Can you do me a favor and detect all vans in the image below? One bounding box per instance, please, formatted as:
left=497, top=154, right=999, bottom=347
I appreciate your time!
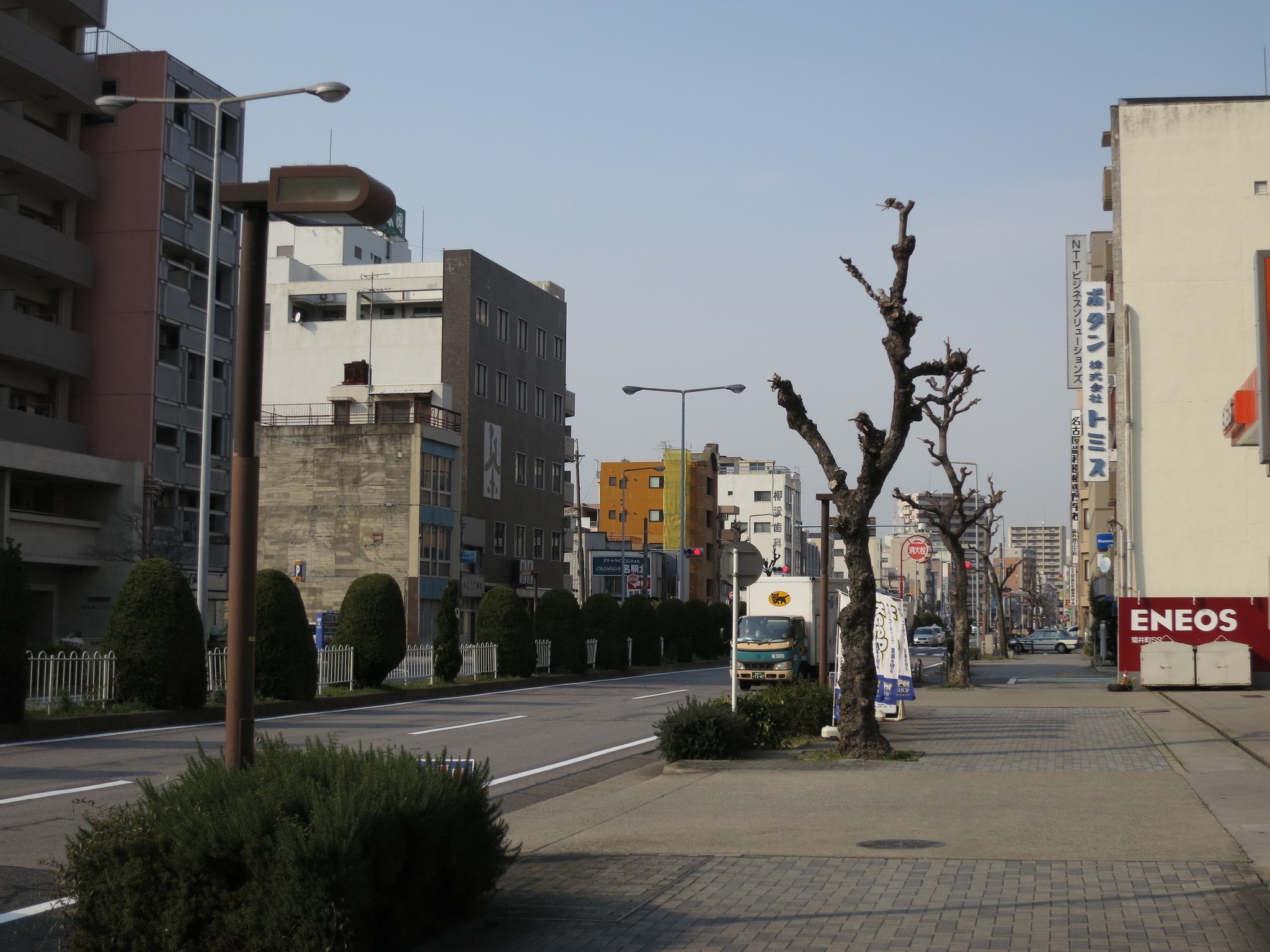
left=970, top=622, right=982, bottom=636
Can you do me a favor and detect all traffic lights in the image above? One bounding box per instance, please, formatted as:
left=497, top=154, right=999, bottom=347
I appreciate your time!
left=954, top=562, right=971, bottom=568
left=684, top=548, right=704, bottom=556
left=772, top=566, right=789, bottom=573
left=905, top=593, right=914, bottom=597
left=888, top=589, right=899, bottom=594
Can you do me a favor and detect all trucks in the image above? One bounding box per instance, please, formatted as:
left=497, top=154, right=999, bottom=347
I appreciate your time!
left=720, top=576, right=851, bottom=690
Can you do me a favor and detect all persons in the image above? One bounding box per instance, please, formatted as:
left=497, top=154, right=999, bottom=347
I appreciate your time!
left=1007, top=624, right=1010, bottom=631
left=1055, top=625, right=1059, bottom=629
left=1027, top=625, right=1033, bottom=636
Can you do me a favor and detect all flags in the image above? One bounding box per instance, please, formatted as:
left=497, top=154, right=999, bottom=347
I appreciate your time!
left=833, top=592, right=916, bottom=720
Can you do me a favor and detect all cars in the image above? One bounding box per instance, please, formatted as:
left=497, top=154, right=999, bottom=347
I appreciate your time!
left=1008, top=625, right=1085, bottom=654
left=992, top=624, right=996, bottom=629
left=913, top=624, right=946, bottom=647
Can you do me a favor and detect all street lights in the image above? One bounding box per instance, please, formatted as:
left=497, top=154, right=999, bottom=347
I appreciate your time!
left=994, top=607, right=998, bottom=631
left=222, top=164, right=396, bottom=770
left=892, top=533, right=940, bottom=615
left=621, top=385, right=746, bottom=603
left=749, top=513, right=780, bottom=543
left=931, top=460, right=980, bottom=660
left=816, top=489, right=856, bottom=690
left=621, top=466, right=666, bottom=604
left=95, top=83, right=351, bottom=651
